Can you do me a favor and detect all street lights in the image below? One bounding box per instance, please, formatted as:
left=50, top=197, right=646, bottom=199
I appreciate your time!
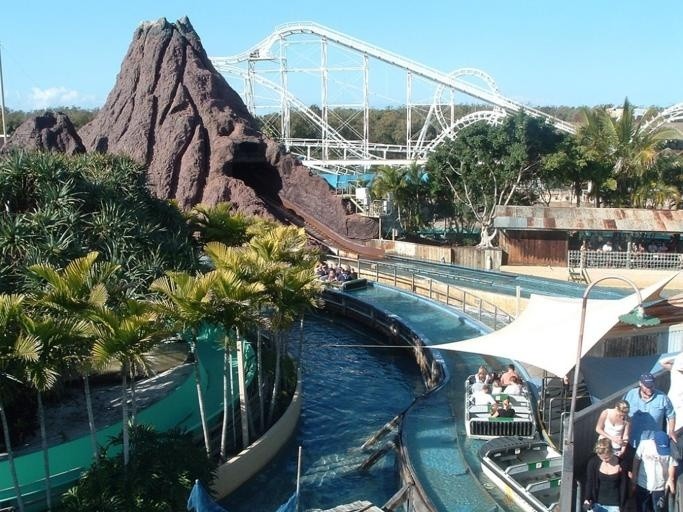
left=558, top=274, right=661, bottom=511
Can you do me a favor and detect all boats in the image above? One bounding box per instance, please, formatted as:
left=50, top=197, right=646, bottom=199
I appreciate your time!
left=476, top=434, right=593, bottom=512
left=465, top=374, right=538, bottom=443
left=316, top=274, right=369, bottom=292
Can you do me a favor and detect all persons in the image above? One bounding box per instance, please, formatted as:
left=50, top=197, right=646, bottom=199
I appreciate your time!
left=472, top=363, right=522, bottom=395
left=581, top=438, right=627, bottom=511
left=594, top=400, right=630, bottom=457
left=580, top=236, right=677, bottom=255
left=629, top=430, right=678, bottom=511
left=319, top=261, right=355, bottom=283
left=491, top=398, right=515, bottom=418
left=620, top=371, right=677, bottom=476
left=469, top=385, right=496, bottom=404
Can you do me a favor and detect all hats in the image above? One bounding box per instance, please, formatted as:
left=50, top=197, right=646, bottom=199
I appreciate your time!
left=654, top=432, right=670, bottom=455
left=640, top=373, right=656, bottom=388
